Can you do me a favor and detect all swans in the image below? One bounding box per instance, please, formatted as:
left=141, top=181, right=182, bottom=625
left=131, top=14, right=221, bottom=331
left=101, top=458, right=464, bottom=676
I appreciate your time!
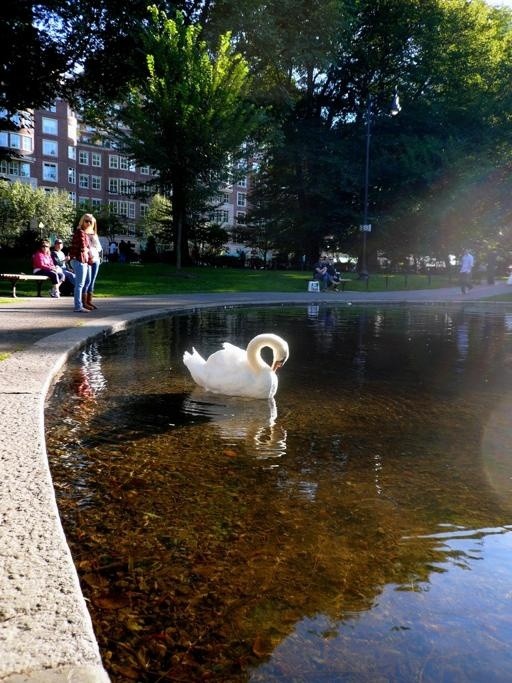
left=182, top=332, right=289, bottom=399
left=182, top=386, right=287, bottom=461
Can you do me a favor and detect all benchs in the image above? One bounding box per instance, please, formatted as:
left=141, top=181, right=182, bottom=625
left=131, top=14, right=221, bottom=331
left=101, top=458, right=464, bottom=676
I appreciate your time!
left=307, top=279, right=352, bottom=291
left=0, top=273, right=50, bottom=297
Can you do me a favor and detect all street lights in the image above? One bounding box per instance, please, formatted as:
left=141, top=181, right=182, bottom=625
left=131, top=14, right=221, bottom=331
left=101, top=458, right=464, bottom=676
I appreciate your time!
left=356, top=81, right=403, bottom=281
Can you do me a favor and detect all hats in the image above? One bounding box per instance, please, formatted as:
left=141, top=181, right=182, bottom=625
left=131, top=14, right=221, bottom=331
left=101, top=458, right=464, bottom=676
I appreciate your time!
left=55, top=239, right=63, bottom=244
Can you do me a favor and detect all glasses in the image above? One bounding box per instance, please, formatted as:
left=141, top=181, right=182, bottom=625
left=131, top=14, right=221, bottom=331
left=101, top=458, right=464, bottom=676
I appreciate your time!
left=84, top=219, right=92, bottom=224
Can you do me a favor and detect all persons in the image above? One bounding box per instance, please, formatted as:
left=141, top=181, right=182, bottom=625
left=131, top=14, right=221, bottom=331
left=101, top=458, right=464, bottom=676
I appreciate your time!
left=459, top=248, right=497, bottom=295
left=32, top=214, right=139, bottom=313
left=313, top=255, right=343, bottom=292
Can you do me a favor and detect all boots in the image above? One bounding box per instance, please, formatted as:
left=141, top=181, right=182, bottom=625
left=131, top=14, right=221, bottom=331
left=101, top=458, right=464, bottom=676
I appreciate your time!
left=82, top=293, right=98, bottom=310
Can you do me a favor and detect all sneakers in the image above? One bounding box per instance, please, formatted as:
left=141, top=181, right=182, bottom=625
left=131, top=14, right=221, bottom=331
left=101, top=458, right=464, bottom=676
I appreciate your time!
left=81, top=309, right=90, bottom=312
left=49, top=290, right=59, bottom=298
